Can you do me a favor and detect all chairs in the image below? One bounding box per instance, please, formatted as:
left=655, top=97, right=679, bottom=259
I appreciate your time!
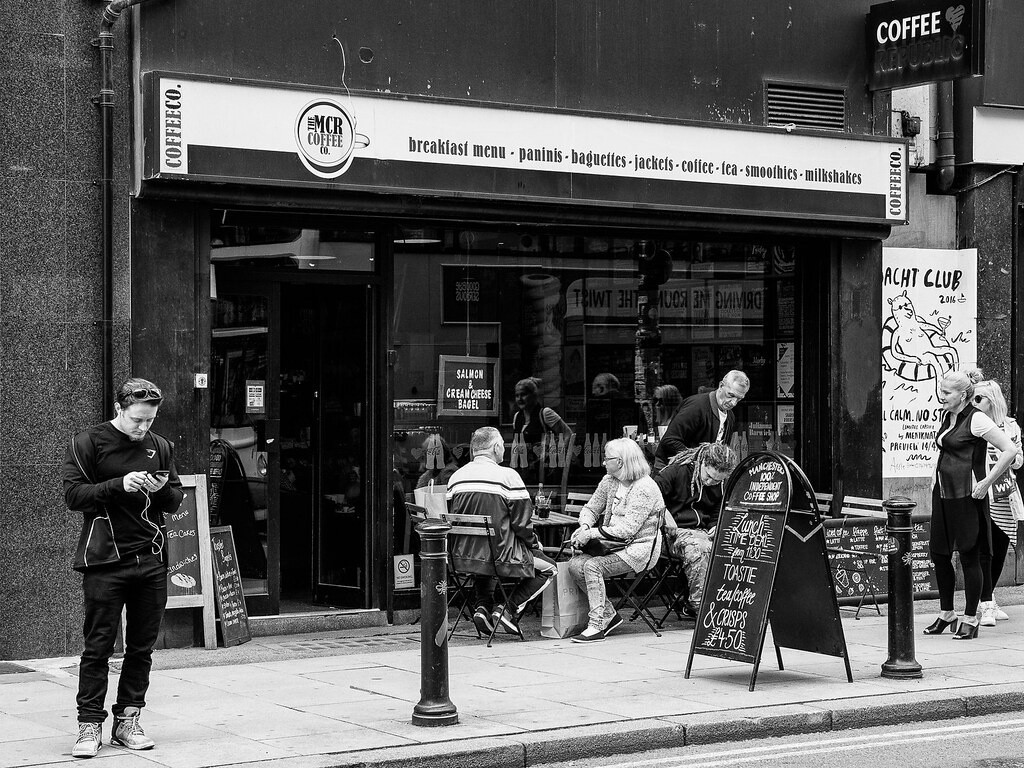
left=405, top=492, right=697, bottom=647
left=807, top=491, right=888, bottom=620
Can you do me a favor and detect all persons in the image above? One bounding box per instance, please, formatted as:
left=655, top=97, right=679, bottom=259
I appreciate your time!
left=510, top=377, right=573, bottom=486
left=586, top=372, right=648, bottom=489
left=451, top=443, right=471, bottom=469
left=62, top=378, right=184, bottom=757
left=972, top=379, right=1024, bottom=625
left=654, top=442, right=736, bottom=616
left=415, top=434, right=458, bottom=490
left=651, top=385, right=683, bottom=426
left=655, top=370, right=750, bottom=482
left=924, top=368, right=1017, bottom=641
left=448, top=426, right=558, bottom=635
left=568, top=437, right=666, bottom=642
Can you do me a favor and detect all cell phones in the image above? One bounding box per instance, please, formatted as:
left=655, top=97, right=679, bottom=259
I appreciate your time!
left=152, top=470, right=170, bottom=481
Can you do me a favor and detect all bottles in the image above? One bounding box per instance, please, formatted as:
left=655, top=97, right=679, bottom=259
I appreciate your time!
left=426, top=433, right=445, bottom=469
left=394, top=402, right=436, bottom=422
left=509, top=432, right=528, bottom=468
left=730, top=430, right=780, bottom=465
left=549, top=433, right=566, bottom=468
left=583, top=433, right=607, bottom=467
left=648, top=428, right=655, bottom=443
left=639, top=433, right=645, bottom=457
left=535, top=481, right=545, bottom=518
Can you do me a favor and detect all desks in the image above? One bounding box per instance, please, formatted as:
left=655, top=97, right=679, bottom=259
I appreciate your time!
left=530, top=509, right=579, bottom=527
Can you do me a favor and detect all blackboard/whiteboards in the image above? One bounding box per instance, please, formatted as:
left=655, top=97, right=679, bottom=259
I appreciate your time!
left=690, top=451, right=849, bottom=664
left=824, top=515, right=956, bottom=607
left=120, top=474, right=214, bottom=609
left=210, top=525, right=251, bottom=649
left=437, top=354, right=500, bottom=417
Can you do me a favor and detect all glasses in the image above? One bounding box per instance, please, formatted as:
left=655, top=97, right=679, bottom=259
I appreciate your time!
left=121, top=388, right=163, bottom=402
left=972, top=395, right=988, bottom=403
left=653, top=398, right=663, bottom=404
left=603, top=457, right=616, bottom=461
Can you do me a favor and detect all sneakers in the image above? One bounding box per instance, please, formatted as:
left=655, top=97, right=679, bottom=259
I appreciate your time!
left=977, top=599, right=996, bottom=625
left=473, top=606, right=495, bottom=636
left=110, top=706, right=155, bottom=749
left=492, top=604, right=523, bottom=634
left=992, top=594, right=1010, bottom=619
left=72, top=722, right=102, bottom=757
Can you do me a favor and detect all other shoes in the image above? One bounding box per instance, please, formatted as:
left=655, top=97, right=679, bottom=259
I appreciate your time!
left=604, top=612, right=623, bottom=637
left=571, top=630, right=606, bottom=642
left=683, top=607, right=698, bottom=620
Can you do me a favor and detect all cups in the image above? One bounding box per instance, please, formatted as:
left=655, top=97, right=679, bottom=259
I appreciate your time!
left=537, top=497, right=552, bottom=521
left=658, top=426, right=668, bottom=441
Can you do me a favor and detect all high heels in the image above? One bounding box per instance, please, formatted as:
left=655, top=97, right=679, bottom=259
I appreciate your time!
left=952, top=622, right=979, bottom=639
left=923, top=617, right=958, bottom=634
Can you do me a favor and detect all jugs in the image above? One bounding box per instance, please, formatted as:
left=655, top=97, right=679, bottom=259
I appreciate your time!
left=623, top=425, right=638, bottom=439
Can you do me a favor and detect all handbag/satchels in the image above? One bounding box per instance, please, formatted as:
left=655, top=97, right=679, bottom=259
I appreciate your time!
left=540, top=540, right=590, bottom=638
left=1008, top=482, right=1024, bottom=522
